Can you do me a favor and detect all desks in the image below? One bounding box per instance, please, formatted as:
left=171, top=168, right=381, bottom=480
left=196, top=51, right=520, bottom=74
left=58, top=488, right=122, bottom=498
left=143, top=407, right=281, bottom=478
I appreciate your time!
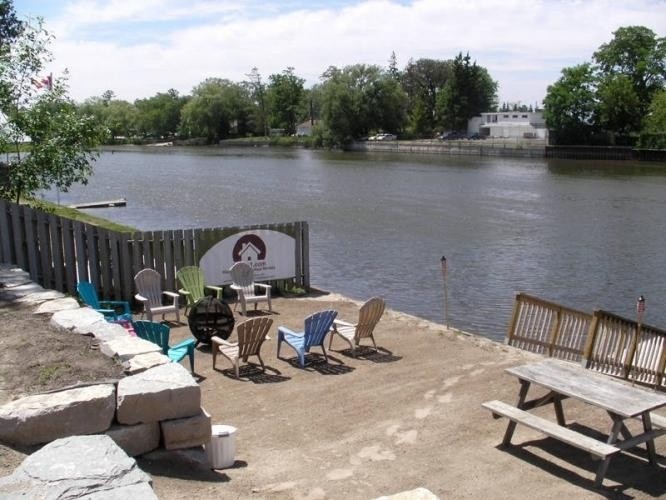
left=503, top=357, right=665, bottom=488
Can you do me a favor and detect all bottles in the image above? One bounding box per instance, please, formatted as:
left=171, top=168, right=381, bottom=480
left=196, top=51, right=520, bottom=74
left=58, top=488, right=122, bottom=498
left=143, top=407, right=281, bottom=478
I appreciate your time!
left=637, top=295, right=645, bottom=312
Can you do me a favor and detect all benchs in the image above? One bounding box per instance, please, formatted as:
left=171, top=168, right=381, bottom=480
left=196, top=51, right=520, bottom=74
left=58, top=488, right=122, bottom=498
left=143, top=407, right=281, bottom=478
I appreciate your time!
left=633, top=413, right=665, bottom=429
left=483, top=401, right=621, bottom=460
